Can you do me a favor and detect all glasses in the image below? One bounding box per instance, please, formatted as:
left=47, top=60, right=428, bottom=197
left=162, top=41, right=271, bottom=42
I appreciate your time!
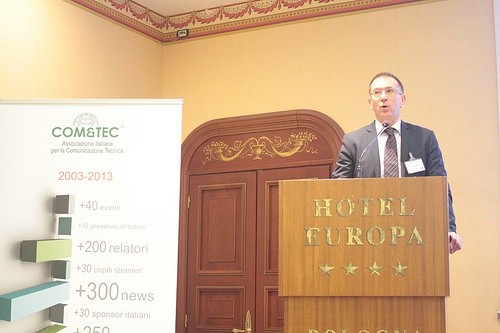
left=370, top=87, right=399, bottom=99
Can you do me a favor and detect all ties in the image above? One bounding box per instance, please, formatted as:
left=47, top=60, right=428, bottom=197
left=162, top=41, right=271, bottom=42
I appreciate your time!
left=384, top=127, right=399, bottom=177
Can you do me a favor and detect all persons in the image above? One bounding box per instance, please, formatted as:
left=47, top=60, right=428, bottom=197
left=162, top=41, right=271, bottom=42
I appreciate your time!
left=332, top=72, right=462, bottom=254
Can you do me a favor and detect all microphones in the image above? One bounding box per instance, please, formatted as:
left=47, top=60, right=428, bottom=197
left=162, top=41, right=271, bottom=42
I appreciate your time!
left=357, top=121, right=389, bottom=178
